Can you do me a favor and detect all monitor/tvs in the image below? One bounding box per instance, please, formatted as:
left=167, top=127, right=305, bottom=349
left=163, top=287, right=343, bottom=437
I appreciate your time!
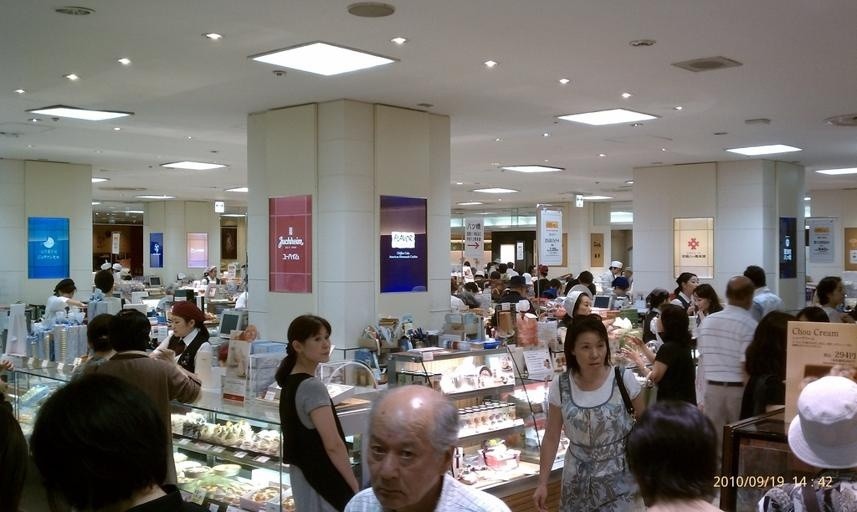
left=149, top=276, right=161, bottom=287
left=218, top=311, right=242, bottom=339
left=592, top=295, right=612, bottom=310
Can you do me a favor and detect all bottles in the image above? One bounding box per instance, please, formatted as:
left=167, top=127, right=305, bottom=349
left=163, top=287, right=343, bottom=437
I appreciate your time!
left=395, top=368, right=441, bottom=393
left=457, top=399, right=517, bottom=434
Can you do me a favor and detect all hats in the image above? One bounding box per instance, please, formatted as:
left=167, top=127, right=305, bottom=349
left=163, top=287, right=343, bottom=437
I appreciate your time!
left=101, top=261, right=112, bottom=271
left=565, top=290, right=587, bottom=319
left=507, top=275, right=529, bottom=286
left=611, top=261, right=622, bottom=269
left=54, top=279, right=76, bottom=291
left=113, top=263, right=122, bottom=271
left=120, top=268, right=132, bottom=277
left=646, top=288, right=669, bottom=303
left=539, top=264, right=549, bottom=273
left=787, top=376, right=857, bottom=471
left=172, top=302, right=207, bottom=321
left=204, top=264, right=217, bottom=274
left=675, top=273, right=697, bottom=285
left=610, top=277, right=630, bottom=289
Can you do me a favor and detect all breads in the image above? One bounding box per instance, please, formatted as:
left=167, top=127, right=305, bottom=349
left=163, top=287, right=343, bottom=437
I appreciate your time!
left=210, top=421, right=284, bottom=457
left=199, top=482, right=295, bottom=512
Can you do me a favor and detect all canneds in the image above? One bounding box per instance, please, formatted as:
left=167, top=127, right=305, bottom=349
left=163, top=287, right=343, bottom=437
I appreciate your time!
left=458, top=399, right=516, bottom=430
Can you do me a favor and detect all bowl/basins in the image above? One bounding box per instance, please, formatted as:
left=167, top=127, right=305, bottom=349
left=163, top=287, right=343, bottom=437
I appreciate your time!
left=184, top=461, right=242, bottom=479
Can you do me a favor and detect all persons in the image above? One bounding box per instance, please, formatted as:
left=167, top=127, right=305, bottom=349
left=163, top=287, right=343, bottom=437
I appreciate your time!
left=273, top=314, right=361, bottom=512
left=0, top=358, right=16, bottom=395
left=0, top=404, right=28, bottom=511
left=26, top=375, right=212, bottom=511
left=91, top=309, right=201, bottom=487
left=200, top=265, right=221, bottom=285
left=147, top=301, right=213, bottom=389
left=100, top=262, right=132, bottom=281
left=341, top=381, right=514, bottom=512
left=90, top=270, right=130, bottom=316
left=67, top=312, right=116, bottom=382
left=620, top=399, right=732, bottom=512
left=621, top=263, right=857, bottom=477
left=755, top=374, right=857, bottom=512
left=530, top=313, right=646, bottom=512
left=450, top=258, right=634, bottom=344
left=154, top=282, right=178, bottom=313
left=44, top=278, right=89, bottom=322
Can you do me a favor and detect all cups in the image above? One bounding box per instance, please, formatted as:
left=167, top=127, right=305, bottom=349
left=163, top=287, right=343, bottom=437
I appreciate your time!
left=358, top=370, right=388, bottom=387
left=398, top=337, right=432, bottom=351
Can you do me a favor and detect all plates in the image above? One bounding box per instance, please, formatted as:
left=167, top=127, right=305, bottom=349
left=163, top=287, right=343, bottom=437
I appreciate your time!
left=437, top=334, right=500, bottom=352
